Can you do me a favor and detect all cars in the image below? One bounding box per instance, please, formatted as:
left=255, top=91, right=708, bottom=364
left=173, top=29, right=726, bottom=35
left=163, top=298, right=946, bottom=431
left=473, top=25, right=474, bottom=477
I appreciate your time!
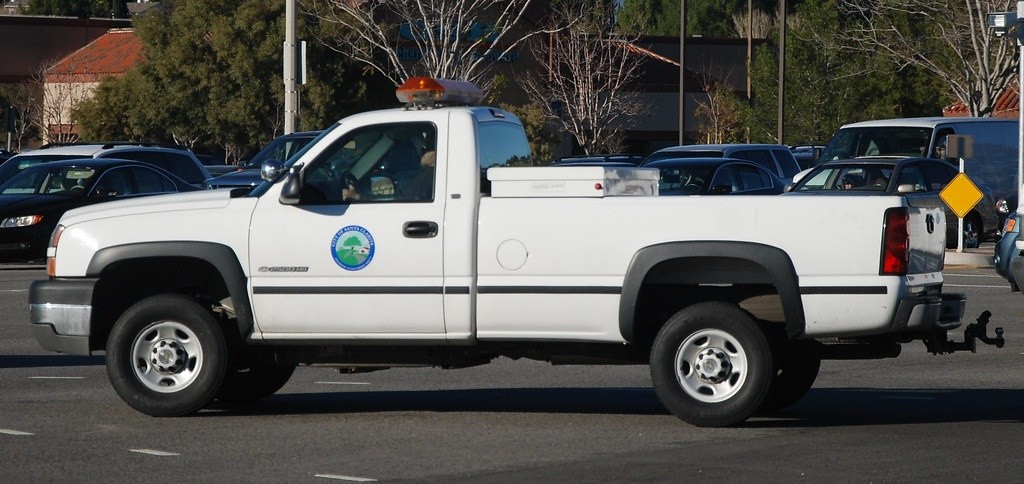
left=0, top=157, right=208, bottom=265
left=549, top=144, right=827, bottom=195
left=201, top=128, right=330, bottom=193
left=780, top=155, right=1000, bottom=249
left=993, top=209, right=1024, bottom=292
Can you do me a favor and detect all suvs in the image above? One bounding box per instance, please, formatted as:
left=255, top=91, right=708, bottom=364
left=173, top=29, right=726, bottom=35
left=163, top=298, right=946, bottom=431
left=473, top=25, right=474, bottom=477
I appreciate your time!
left=0, top=140, right=214, bottom=191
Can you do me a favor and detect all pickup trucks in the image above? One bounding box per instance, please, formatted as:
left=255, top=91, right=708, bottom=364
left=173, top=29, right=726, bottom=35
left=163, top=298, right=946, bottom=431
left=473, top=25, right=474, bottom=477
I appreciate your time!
left=26, top=76, right=1001, bottom=428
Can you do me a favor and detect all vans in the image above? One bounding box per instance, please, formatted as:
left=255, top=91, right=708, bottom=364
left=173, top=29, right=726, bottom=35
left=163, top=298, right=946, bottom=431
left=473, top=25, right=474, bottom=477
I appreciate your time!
left=808, top=116, right=1019, bottom=243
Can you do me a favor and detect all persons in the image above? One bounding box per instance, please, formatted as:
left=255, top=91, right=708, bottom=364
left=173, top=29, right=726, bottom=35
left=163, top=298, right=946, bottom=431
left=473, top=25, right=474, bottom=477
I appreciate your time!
left=341, top=132, right=436, bottom=200
left=841, top=174, right=856, bottom=189
left=920, top=140, right=946, bottom=156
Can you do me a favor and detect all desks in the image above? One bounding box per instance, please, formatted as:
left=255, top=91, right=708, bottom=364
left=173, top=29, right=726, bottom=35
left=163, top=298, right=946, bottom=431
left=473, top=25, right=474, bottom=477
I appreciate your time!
left=486, top=167, right=661, bottom=197
